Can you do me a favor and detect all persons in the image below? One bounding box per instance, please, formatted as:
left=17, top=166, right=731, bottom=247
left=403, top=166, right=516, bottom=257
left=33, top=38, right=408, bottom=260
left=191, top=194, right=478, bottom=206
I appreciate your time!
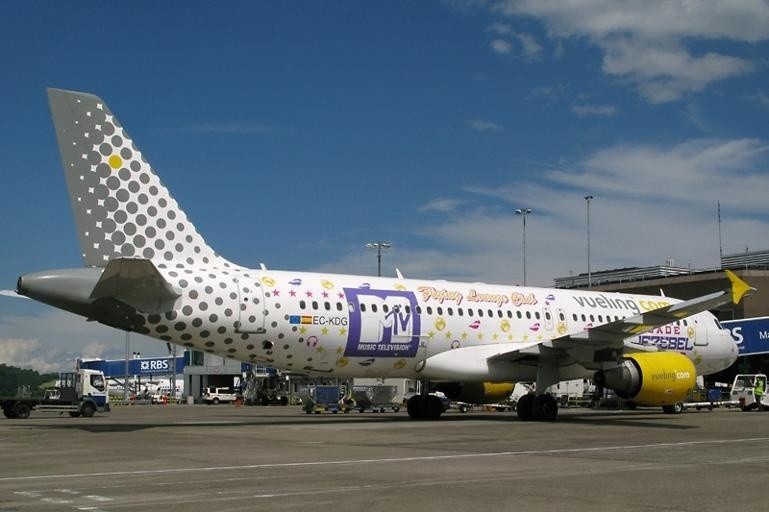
left=749, top=377, right=764, bottom=411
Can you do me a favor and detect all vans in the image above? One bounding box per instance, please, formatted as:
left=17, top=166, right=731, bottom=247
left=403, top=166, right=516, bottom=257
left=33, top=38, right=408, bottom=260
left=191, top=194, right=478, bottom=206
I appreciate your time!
left=202, top=387, right=238, bottom=404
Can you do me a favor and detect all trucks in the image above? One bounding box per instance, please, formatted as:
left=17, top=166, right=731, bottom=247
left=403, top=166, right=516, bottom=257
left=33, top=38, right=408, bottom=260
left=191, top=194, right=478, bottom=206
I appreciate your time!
left=1, top=369, right=108, bottom=419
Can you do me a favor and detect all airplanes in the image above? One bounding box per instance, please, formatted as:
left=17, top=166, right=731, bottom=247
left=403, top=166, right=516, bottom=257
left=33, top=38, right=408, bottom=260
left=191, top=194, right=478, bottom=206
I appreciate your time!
left=16, top=87, right=758, bottom=421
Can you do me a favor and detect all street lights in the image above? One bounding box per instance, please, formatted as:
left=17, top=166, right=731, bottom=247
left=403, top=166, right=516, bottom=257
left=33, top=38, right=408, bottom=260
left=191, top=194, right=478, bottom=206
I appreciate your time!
left=365, top=240, right=393, bottom=277
left=515, top=207, right=531, bottom=286
left=584, top=196, right=594, bottom=290
